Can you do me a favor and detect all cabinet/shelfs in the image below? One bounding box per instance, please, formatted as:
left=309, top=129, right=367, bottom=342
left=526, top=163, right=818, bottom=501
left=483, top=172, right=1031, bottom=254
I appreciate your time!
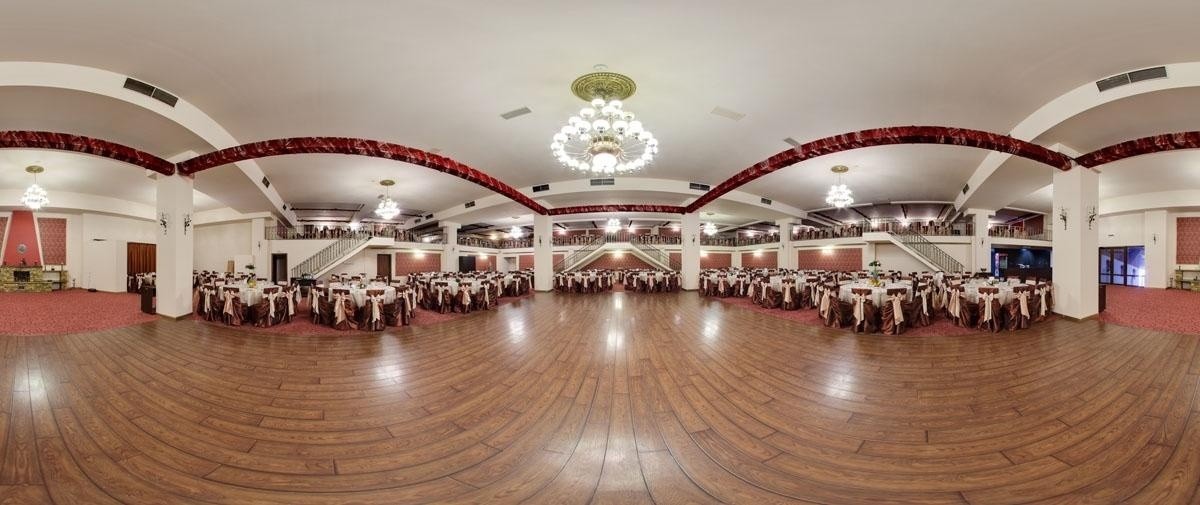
left=141, top=286, right=156, bottom=315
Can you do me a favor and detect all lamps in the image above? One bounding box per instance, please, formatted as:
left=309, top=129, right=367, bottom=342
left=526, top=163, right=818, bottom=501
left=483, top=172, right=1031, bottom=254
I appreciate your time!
left=825, top=165, right=854, bottom=208
left=550, top=73, right=658, bottom=176
left=374, top=180, right=401, bottom=220
left=21, top=166, right=49, bottom=209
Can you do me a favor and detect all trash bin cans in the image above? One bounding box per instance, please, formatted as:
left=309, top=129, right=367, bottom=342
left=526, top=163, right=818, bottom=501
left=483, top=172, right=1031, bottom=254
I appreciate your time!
left=1100, top=284, right=1106, bottom=312
